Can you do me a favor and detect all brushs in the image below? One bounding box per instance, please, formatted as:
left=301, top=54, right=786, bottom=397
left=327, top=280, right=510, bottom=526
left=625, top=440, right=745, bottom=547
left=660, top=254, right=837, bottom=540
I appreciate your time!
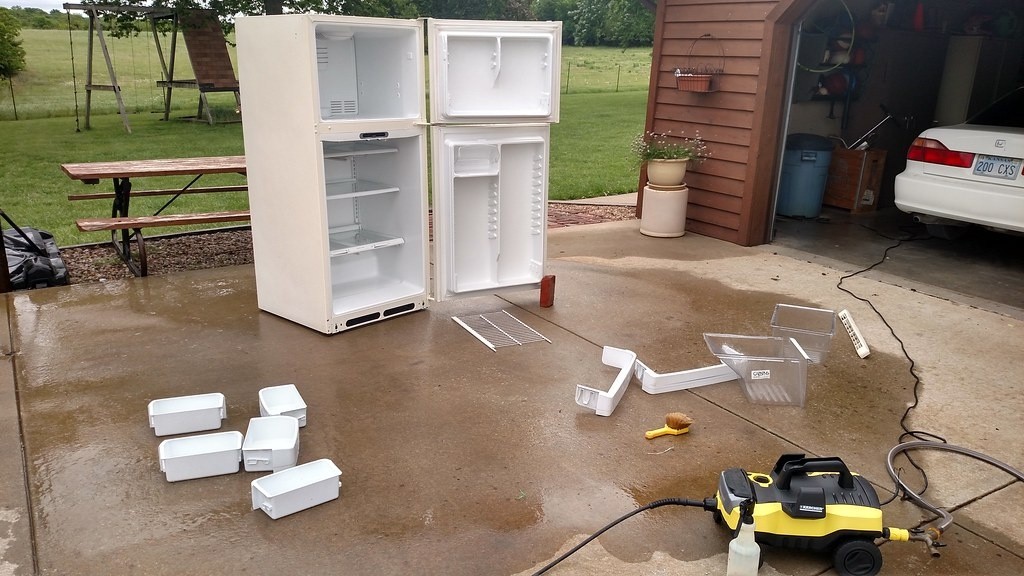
left=644, top=412, right=693, bottom=440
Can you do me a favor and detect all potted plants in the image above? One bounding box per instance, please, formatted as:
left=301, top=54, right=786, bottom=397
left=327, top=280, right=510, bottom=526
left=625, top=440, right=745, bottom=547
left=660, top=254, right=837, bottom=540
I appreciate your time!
left=646, top=158, right=693, bottom=186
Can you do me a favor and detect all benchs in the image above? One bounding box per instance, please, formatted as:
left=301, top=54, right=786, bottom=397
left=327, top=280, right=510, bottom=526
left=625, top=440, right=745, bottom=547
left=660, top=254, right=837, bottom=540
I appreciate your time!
left=68, top=184, right=250, bottom=232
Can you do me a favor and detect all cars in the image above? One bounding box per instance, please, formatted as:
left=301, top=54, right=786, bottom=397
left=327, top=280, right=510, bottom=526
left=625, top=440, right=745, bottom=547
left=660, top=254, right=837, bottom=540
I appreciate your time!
left=894, top=83, right=1024, bottom=242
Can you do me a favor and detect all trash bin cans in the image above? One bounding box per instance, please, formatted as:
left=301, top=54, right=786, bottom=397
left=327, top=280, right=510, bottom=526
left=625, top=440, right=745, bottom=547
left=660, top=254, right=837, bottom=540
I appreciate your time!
left=775, top=133, right=836, bottom=219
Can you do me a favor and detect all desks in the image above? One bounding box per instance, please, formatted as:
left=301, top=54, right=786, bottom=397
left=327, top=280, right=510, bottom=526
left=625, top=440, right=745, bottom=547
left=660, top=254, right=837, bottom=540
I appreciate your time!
left=60, top=155, right=247, bottom=277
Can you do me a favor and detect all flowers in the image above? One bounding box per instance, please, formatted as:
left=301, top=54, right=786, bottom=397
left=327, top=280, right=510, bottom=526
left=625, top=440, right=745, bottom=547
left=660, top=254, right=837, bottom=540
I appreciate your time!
left=625, top=127, right=713, bottom=173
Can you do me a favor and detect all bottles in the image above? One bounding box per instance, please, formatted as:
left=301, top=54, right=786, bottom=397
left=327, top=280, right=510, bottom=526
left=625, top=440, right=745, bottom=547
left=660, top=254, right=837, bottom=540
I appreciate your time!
left=725, top=498, right=760, bottom=576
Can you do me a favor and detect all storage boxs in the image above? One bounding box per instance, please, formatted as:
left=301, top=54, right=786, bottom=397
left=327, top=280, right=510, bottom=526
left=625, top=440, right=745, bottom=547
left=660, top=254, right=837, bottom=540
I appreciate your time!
left=702, top=308, right=836, bottom=407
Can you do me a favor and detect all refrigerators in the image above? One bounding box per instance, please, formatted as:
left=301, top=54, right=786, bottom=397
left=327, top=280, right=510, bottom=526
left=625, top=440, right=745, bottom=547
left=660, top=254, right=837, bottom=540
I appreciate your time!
left=234, top=14, right=563, bottom=333
